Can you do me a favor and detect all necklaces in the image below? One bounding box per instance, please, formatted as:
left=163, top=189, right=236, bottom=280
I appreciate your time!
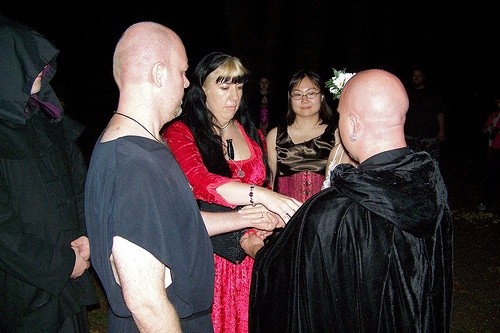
left=227, top=145, right=245, bottom=178
left=260, top=90, right=268, bottom=95
left=221, top=120, right=230, bottom=131
left=113, top=111, right=194, bottom=192
left=293, top=119, right=319, bottom=142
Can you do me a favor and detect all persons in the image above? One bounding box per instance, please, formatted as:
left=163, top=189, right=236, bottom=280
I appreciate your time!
left=84, top=21, right=285, bottom=333
left=475, top=97, right=500, bottom=211
left=0, top=24, right=100, bottom=333
left=248, top=68, right=454, bottom=333
left=162, top=52, right=304, bottom=333
left=252, top=70, right=359, bottom=203
left=404, top=70, right=446, bottom=160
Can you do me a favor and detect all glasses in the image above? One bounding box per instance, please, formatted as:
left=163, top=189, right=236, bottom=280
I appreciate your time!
left=289, top=88, right=321, bottom=100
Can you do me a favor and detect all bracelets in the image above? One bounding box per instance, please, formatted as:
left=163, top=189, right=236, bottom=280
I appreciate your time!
left=249, top=184, right=254, bottom=204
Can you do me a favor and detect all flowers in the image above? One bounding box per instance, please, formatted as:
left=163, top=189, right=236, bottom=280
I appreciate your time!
left=325, top=68, right=356, bottom=100
